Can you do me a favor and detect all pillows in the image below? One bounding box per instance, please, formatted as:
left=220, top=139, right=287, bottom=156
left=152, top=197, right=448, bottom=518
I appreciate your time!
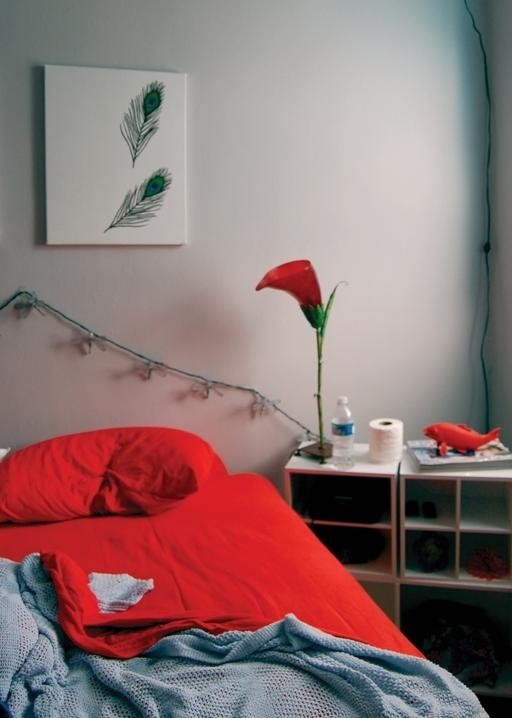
left=0, top=426, right=231, bottom=524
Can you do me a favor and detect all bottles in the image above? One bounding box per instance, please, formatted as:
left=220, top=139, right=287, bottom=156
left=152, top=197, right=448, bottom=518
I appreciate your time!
left=331, top=396, right=354, bottom=469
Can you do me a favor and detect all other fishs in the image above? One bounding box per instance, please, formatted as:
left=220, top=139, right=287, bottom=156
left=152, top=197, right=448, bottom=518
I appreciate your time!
left=422, top=423, right=501, bottom=456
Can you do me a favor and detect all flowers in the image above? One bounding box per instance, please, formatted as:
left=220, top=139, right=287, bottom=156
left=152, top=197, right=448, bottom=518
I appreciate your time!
left=256, top=260, right=348, bottom=449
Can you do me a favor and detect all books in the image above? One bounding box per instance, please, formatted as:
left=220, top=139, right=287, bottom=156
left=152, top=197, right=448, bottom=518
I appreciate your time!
left=404, top=435, right=512, bottom=474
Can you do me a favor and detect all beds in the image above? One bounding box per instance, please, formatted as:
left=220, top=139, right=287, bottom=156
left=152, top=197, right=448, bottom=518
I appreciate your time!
left=1, top=472, right=486, bottom=717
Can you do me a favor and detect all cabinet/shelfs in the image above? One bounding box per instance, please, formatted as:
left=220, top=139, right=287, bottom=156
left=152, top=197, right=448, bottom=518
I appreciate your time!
left=400, top=440, right=512, bottom=583
left=284, top=437, right=399, bottom=582
left=356, top=580, right=511, bottom=698
